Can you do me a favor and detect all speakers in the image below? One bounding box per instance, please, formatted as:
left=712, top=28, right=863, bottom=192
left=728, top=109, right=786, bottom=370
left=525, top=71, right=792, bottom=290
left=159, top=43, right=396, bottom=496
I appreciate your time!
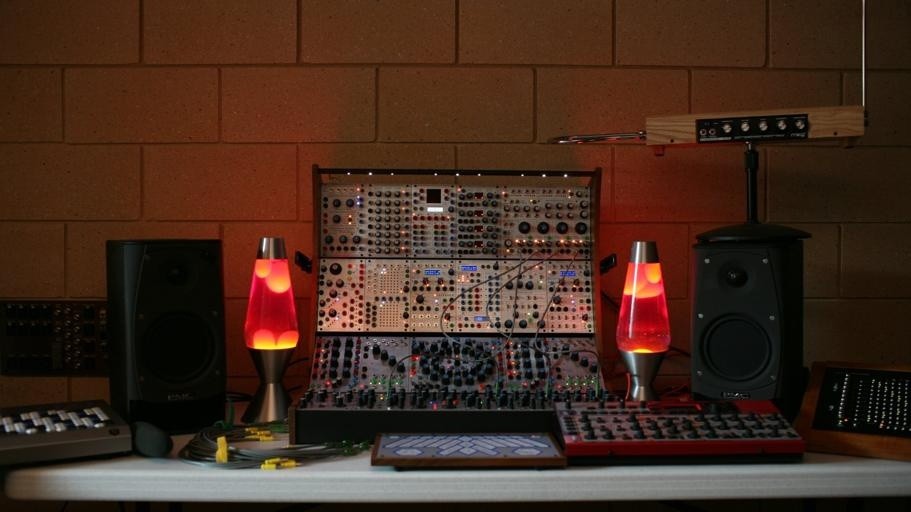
left=689, top=245, right=804, bottom=403
left=105, top=238, right=226, bottom=434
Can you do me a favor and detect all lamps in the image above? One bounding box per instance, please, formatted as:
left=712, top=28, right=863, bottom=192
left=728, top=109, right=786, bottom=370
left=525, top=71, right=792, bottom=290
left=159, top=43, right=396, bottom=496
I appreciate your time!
left=243, top=236, right=301, bottom=426
left=614, top=240, right=672, bottom=402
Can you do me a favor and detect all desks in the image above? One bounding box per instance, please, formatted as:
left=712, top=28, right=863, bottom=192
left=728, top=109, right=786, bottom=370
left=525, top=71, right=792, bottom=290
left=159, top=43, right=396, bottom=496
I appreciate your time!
left=6, top=390, right=911, bottom=503
